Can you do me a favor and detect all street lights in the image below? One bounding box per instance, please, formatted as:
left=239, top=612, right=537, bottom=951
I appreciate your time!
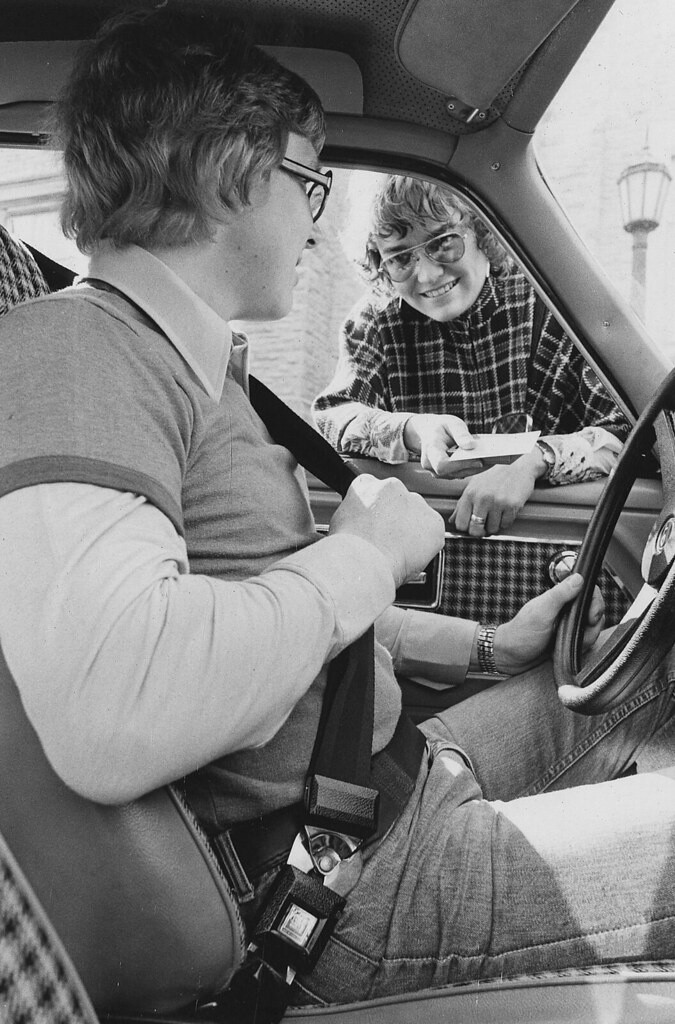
left=617, top=123, right=674, bottom=330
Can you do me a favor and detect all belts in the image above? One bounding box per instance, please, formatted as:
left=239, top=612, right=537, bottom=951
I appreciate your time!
left=209, top=753, right=389, bottom=890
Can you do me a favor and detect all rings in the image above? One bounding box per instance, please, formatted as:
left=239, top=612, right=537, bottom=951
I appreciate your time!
left=470, top=514, right=485, bottom=524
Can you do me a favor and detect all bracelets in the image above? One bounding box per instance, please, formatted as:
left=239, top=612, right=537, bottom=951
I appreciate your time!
left=477, top=624, right=499, bottom=676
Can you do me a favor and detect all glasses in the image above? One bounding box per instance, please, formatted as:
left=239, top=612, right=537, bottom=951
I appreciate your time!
left=376, top=223, right=474, bottom=283
left=280, top=156, right=335, bottom=224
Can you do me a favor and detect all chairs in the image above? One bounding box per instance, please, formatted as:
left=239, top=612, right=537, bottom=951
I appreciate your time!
left=0, top=225, right=675, bottom=1023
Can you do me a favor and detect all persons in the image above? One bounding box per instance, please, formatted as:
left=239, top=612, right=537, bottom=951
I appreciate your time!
left=0, top=12, right=675, bottom=1006
